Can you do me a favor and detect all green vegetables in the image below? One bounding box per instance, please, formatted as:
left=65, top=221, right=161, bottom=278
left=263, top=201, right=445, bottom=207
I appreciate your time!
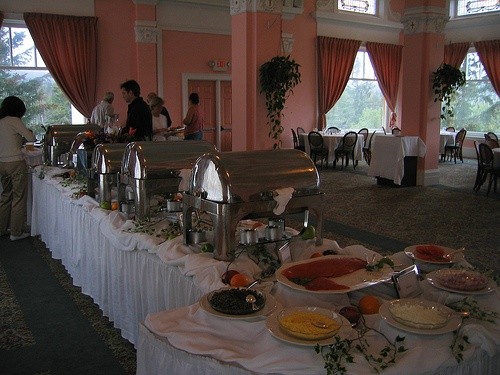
left=288, top=278, right=312, bottom=285
left=364, top=257, right=394, bottom=271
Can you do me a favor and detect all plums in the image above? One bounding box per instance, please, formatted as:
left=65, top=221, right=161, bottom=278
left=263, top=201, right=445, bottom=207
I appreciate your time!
left=323, top=250, right=337, bottom=255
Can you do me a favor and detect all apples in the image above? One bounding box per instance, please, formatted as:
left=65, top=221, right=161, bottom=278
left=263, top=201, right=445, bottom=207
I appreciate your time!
left=310, top=252, right=324, bottom=258
left=173, top=196, right=182, bottom=200
left=339, top=295, right=379, bottom=324
left=221, top=270, right=251, bottom=288
left=201, top=243, right=214, bottom=253
left=80, top=192, right=86, bottom=196
left=251, top=222, right=266, bottom=230
left=99, top=201, right=118, bottom=211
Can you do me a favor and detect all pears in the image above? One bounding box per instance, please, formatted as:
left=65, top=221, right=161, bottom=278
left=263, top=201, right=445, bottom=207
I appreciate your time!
left=301, top=225, right=315, bottom=239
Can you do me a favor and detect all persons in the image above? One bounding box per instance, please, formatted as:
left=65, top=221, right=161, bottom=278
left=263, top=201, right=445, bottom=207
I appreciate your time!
left=146, top=92, right=172, bottom=132
left=90, top=90, right=114, bottom=128
left=115, top=80, right=153, bottom=142
left=183, top=93, right=204, bottom=132
left=0, top=96, right=36, bottom=240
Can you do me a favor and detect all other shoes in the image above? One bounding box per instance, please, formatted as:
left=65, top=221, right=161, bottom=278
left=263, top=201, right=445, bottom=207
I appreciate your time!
left=10, top=233, right=28, bottom=241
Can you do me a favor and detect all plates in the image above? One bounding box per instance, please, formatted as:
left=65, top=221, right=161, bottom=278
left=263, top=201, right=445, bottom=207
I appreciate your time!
left=275, top=253, right=394, bottom=293
left=379, top=297, right=462, bottom=334
left=199, top=289, right=278, bottom=319
left=404, top=245, right=464, bottom=263
left=435, top=270, right=489, bottom=291
left=426, top=268, right=497, bottom=294
left=265, top=306, right=351, bottom=346
left=276, top=307, right=343, bottom=340
left=387, top=298, right=452, bottom=328
left=207, top=286, right=265, bottom=315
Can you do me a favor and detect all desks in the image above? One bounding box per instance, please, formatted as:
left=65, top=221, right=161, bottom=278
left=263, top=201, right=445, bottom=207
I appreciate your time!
left=490, top=147, right=500, bottom=194
left=22, top=150, right=339, bottom=353
left=369, top=134, right=427, bottom=188
left=136, top=252, right=500, bottom=375
left=299, top=132, right=365, bottom=166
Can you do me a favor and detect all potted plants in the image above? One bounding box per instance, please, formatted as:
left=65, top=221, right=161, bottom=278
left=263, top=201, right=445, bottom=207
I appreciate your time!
left=432, top=63, right=467, bottom=119
left=258, top=55, right=302, bottom=149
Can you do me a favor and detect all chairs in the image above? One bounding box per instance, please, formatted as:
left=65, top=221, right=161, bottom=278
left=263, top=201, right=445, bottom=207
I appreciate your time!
left=290, top=126, right=378, bottom=177
left=441, top=127, right=467, bottom=164
left=473, top=132, right=500, bottom=196
left=440, top=132, right=456, bottom=160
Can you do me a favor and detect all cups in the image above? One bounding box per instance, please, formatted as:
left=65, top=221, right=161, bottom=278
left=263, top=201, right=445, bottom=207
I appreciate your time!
left=26, top=142, right=34, bottom=152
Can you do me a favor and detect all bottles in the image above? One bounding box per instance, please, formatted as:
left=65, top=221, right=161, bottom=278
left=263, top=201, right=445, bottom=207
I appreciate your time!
left=167, top=199, right=179, bottom=213
left=240, top=229, right=257, bottom=245
left=265, top=226, right=279, bottom=241
left=269, top=218, right=284, bottom=233
